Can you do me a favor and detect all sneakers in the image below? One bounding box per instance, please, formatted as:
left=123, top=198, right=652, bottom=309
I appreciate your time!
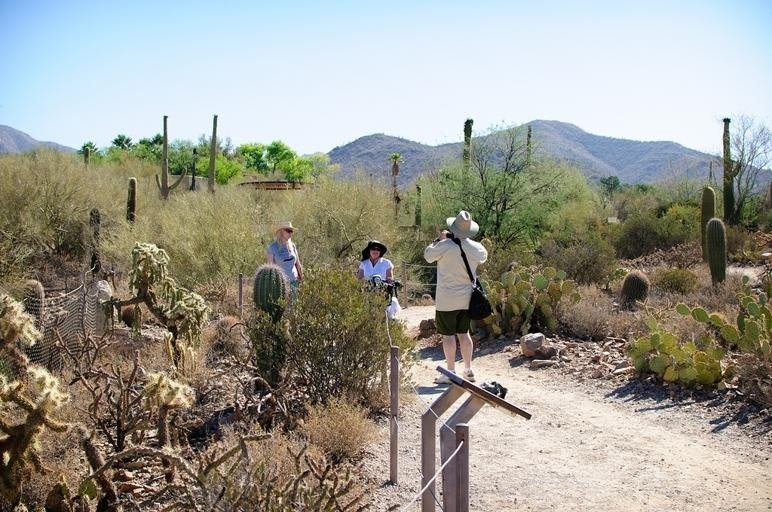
left=435, top=371, right=456, bottom=384
left=461, top=369, right=476, bottom=382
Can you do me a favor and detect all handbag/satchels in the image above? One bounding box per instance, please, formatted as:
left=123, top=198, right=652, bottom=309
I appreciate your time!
left=468, top=289, right=492, bottom=320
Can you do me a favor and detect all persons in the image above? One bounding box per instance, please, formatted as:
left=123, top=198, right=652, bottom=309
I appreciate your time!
left=356, top=240, right=403, bottom=323
left=423, top=210, right=489, bottom=386
left=267, top=220, right=306, bottom=315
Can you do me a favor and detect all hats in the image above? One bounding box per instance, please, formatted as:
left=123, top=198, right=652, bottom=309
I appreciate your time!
left=361, top=239, right=387, bottom=258
left=274, top=221, right=298, bottom=233
left=446, top=211, right=480, bottom=239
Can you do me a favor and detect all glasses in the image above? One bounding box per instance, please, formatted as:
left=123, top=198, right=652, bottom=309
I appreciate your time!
left=371, top=248, right=381, bottom=251
left=285, top=229, right=293, bottom=233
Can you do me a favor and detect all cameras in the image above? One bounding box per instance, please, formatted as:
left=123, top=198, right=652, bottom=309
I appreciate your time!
left=446, top=232, right=454, bottom=239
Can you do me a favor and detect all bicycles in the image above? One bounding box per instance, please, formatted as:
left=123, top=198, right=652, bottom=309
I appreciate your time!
left=367, top=275, right=402, bottom=306
